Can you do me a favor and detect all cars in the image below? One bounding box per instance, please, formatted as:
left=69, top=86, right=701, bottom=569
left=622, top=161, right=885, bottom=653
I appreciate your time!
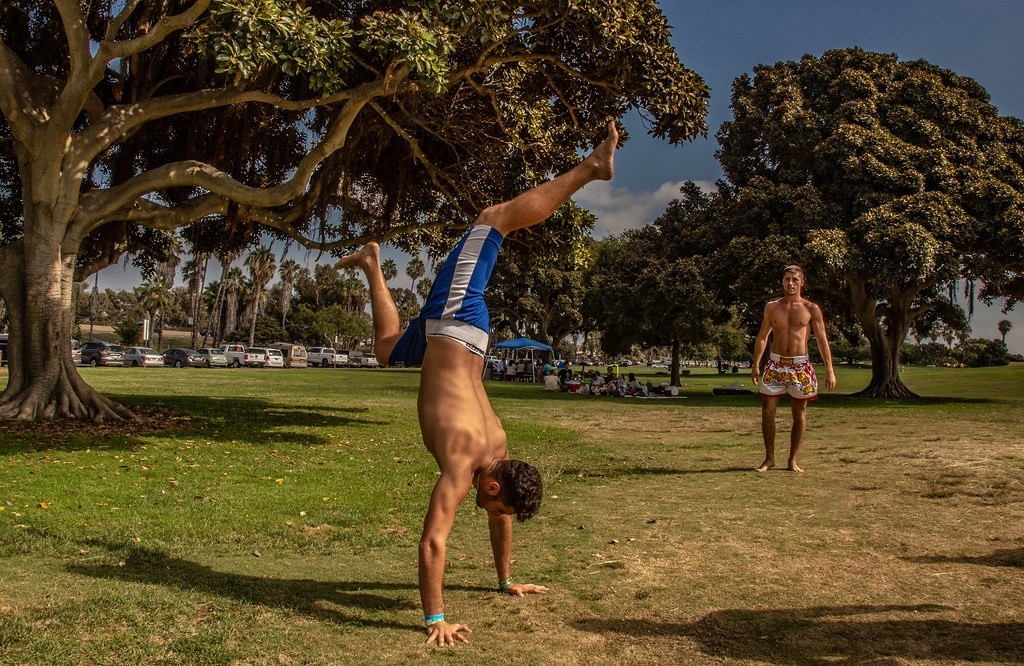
left=162, top=348, right=228, bottom=368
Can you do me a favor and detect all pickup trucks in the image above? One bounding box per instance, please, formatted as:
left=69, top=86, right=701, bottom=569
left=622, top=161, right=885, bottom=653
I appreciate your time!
left=307, top=347, right=379, bottom=368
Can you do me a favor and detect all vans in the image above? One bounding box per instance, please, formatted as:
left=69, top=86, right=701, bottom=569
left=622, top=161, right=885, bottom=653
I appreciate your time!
left=218, top=343, right=309, bottom=369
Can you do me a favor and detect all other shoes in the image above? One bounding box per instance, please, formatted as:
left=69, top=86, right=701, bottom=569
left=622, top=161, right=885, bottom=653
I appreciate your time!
left=600, top=390, right=608, bottom=396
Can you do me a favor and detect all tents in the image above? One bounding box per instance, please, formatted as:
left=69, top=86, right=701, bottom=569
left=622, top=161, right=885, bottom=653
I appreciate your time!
left=483, top=337, right=557, bottom=383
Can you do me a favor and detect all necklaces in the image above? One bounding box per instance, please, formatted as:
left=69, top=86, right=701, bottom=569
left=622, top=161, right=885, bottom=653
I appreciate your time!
left=476, top=459, right=506, bottom=490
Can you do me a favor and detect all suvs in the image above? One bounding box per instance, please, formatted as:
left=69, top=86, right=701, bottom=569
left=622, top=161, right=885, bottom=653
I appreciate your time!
left=70, top=340, right=165, bottom=369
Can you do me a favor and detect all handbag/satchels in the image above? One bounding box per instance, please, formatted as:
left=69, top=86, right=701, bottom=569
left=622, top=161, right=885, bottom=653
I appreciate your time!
left=664, top=385, right=679, bottom=396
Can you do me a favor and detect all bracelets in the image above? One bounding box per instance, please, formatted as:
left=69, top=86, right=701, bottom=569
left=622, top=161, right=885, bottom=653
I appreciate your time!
left=499, top=577, right=513, bottom=587
left=424, top=612, right=445, bottom=625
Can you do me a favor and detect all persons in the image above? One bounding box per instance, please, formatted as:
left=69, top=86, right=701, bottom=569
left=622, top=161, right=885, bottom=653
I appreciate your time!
left=333, top=119, right=619, bottom=645
left=751, top=266, right=836, bottom=473
left=496, top=356, right=640, bottom=396
